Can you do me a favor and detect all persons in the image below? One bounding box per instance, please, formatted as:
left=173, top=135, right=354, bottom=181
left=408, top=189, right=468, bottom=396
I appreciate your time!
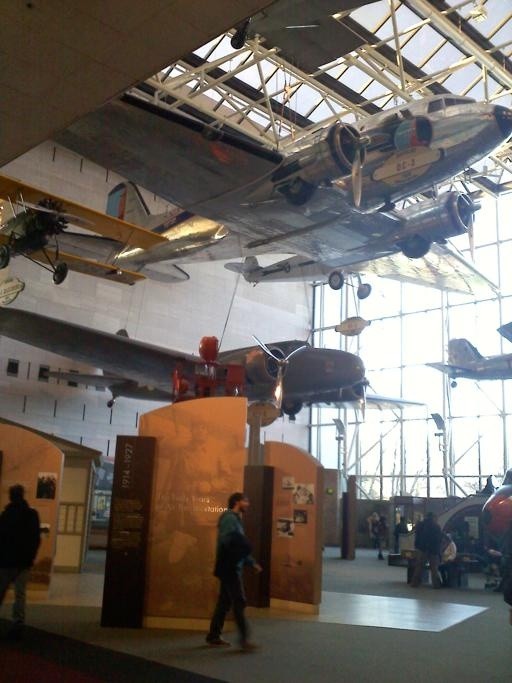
left=367, top=512, right=457, bottom=589
left=1, top=484, right=41, bottom=637
left=205, top=492, right=262, bottom=653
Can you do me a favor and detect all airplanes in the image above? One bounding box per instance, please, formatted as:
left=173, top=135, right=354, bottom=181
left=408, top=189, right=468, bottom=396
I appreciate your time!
left=0, top=170, right=171, bottom=302
left=425, top=321, right=512, bottom=379
left=0, top=306, right=425, bottom=427
left=0, top=92, right=511, bottom=308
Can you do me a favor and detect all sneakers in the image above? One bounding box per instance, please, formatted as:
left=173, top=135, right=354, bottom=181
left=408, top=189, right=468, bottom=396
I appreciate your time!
left=240, top=644, right=261, bottom=652
left=208, top=640, right=231, bottom=648
